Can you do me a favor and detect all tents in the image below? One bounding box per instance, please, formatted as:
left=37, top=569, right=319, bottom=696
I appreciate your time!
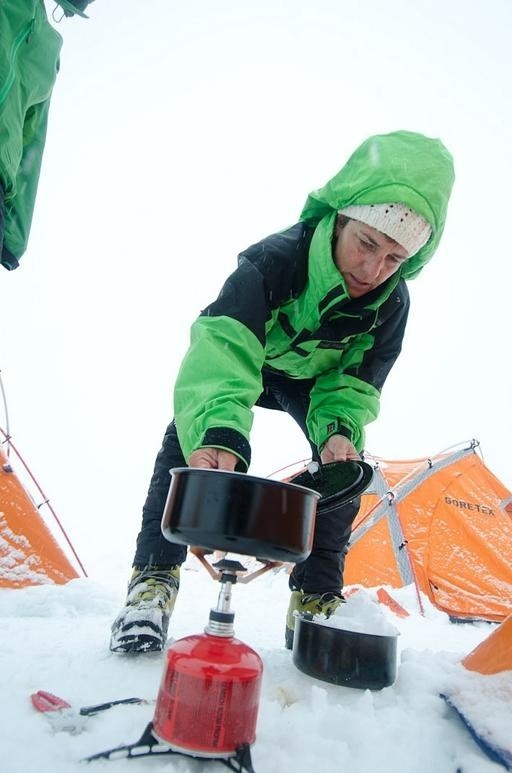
left=438, top=613, right=510, bottom=773
left=344, top=438, right=511, bottom=623
left=0, top=428, right=90, bottom=589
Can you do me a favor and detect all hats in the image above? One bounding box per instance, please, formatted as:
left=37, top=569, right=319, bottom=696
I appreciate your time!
left=332, top=187, right=434, bottom=258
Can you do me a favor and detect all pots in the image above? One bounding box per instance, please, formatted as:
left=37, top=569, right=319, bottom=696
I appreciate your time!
left=160, top=467, right=323, bottom=563
left=291, top=613, right=399, bottom=690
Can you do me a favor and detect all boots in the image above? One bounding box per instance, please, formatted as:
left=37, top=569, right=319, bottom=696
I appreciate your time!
left=284, top=582, right=349, bottom=655
left=107, top=559, right=181, bottom=659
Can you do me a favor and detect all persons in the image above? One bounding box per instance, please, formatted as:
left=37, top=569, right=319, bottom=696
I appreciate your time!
left=0, top=0, right=92, bottom=270
left=108, top=129, right=456, bottom=654
